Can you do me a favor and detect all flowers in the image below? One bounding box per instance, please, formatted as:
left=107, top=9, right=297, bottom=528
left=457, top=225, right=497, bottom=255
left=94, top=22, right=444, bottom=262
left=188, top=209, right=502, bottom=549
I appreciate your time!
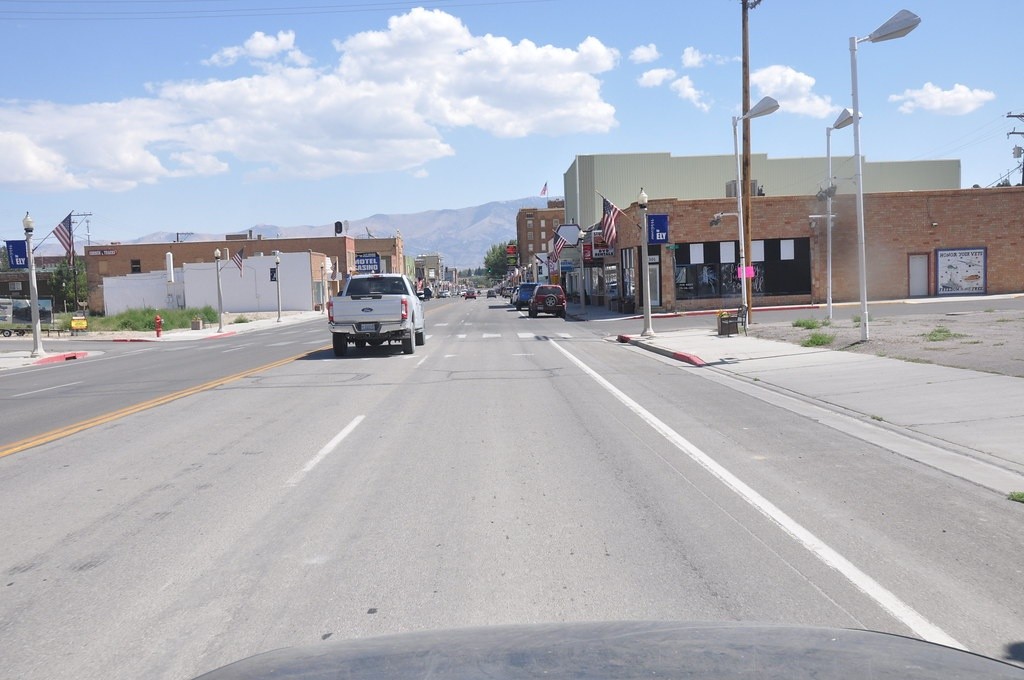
left=192, top=316, right=202, bottom=320
left=717, top=310, right=732, bottom=318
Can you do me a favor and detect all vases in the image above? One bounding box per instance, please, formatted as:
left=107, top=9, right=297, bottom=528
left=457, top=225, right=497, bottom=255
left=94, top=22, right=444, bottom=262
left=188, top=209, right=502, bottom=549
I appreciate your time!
left=191, top=320, right=202, bottom=330
left=718, top=317, right=738, bottom=335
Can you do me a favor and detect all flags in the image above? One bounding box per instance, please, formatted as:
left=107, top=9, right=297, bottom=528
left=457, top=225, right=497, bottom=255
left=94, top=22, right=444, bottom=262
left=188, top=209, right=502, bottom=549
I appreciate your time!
left=232, top=249, right=242, bottom=278
left=52, top=213, right=73, bottom=264
left=330, top=263, right=336, bottom=279
left=552, top=233, right=566, bottom=263
left=602, top=197, right=621, bottom=249
left=541, top=183, right=546, bottom=195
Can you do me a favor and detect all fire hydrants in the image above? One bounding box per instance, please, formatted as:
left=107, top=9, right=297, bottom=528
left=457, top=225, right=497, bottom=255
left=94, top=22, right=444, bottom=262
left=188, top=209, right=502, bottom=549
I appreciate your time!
left=154, top=315, right=164, bottom=337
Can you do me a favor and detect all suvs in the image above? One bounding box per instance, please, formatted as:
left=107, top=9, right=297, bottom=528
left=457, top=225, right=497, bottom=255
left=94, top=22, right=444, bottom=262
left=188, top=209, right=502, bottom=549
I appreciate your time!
left=526, top=285, right=567, bottom=318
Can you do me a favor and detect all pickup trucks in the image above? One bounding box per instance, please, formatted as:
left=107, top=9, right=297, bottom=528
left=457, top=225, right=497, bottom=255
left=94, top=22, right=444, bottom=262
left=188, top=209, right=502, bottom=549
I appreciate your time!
left=325, top=272, right=426, bottom=357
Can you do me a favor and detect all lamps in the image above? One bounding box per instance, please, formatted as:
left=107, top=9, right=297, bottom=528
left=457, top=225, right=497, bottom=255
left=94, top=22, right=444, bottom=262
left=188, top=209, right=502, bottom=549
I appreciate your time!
left=932, top=223, right=939, bottom=228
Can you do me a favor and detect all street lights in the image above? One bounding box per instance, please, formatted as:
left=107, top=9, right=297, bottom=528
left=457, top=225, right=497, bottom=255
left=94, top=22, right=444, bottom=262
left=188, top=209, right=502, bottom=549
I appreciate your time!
left=273, top=250, right=283, bottom=322
left=547, top=255, right=551, bottom=285
left=319, top=261, right=326, bottom=314
left=847, top=8, right=922, bottom=341
left=22, top=210, right=46, bottom=358
left=721, top=95, right=780, bottom=328
left=214, top=247, right=225, bottom=333
left=577, top=228, right=588, bottom=315
left=825, top=106, right=864, bottom=322
left=637, top=187, right=655, bottom=336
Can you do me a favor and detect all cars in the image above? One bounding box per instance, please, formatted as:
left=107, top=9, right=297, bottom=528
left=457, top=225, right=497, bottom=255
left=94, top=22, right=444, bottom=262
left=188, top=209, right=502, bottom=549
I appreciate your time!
left=437, top=288, right=497, bottom=300
left=499, top=283, right=542, bottom=311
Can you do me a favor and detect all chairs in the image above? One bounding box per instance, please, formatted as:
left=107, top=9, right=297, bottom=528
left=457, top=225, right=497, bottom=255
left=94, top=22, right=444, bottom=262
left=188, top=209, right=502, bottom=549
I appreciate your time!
left=721, top=304, right=748, bottom=337
